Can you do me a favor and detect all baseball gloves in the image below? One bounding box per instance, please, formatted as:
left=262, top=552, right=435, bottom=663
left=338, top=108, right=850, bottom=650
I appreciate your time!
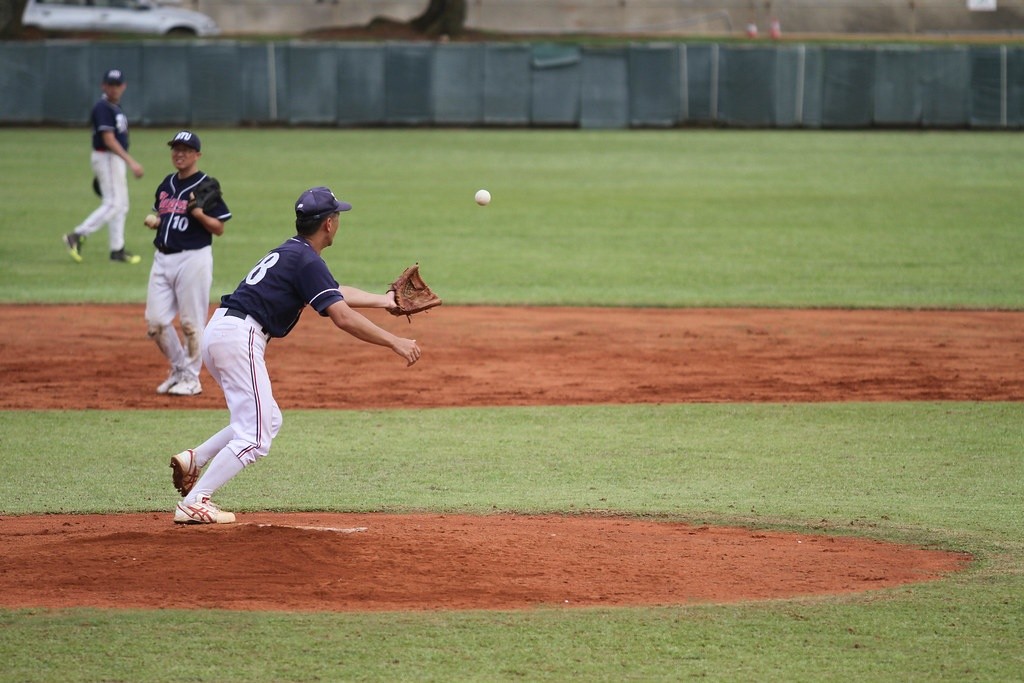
left=385, top=262, right=443, bottom=318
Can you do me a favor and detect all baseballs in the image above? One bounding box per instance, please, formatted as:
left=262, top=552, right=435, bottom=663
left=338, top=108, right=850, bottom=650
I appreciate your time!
left=146, top=214, right=157, bottom=226
left=475, top=189, right=491, bottom=206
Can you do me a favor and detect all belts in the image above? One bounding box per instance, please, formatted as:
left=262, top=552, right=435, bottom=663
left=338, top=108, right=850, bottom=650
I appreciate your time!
left=225, top=309, right=272, bottom=343
left=159, top=248, right=182, bottom=254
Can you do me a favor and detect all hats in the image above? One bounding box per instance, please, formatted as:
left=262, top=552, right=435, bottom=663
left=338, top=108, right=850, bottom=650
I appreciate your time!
left=104, top=68, right=124, bottom=83
left=295, top=187, right=352, bottom=220
left=168, top=130, right=201, bottom=151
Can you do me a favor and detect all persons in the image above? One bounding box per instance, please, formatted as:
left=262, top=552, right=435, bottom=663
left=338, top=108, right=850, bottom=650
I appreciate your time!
left=63, top=67, right=143, bottom=266
left=144, top=130, right=232, bottom=399
left=169, top=187, right=442, bottom=524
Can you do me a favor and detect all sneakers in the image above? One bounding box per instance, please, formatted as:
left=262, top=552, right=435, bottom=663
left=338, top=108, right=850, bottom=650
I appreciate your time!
left=64, top=233, right=83, bottom=261
left=156, top=379, right=177, bottom=394
left=112, top=251, right=140, bottom=263
left=169, top=449, right=200, bottom=497
left=168, top=380, right=202, bottom=394
left=172, top=494, right=235, bottom=525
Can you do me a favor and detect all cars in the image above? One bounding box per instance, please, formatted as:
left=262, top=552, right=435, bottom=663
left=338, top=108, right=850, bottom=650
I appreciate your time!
left=18, top=0, right=222, bottom=40
